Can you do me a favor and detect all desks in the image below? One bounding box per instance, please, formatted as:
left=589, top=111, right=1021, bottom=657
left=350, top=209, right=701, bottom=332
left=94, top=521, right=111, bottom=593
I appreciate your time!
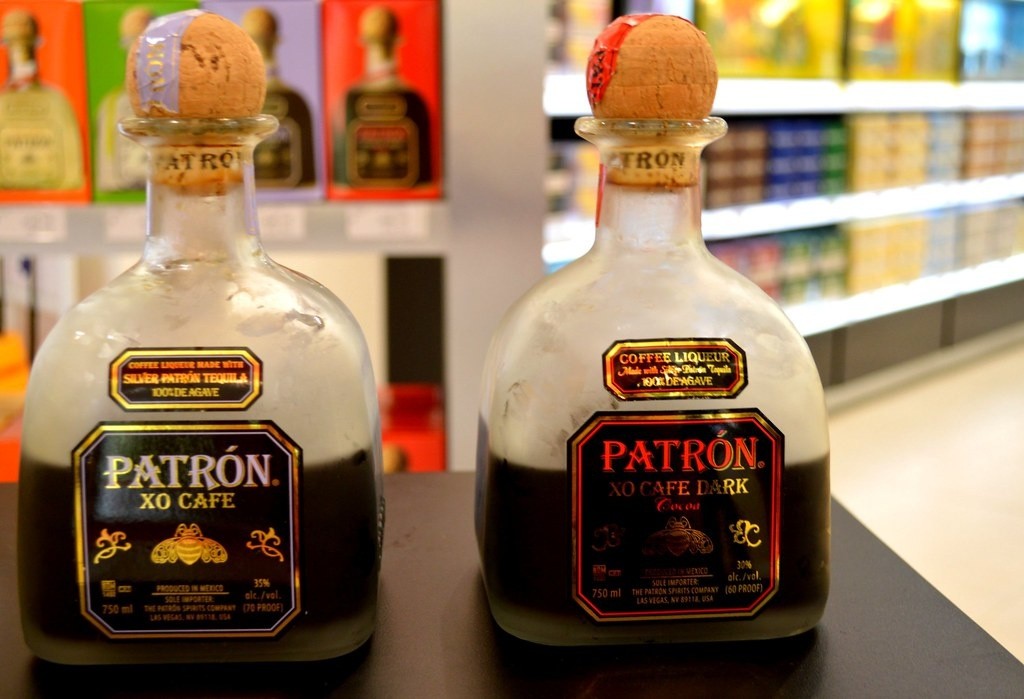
left=0, top=471, right=1024, bottom=699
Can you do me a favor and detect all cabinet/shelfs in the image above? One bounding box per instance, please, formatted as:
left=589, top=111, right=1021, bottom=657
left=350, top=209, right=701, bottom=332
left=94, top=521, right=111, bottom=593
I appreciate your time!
left=542, top=72, right=1024, bottom=413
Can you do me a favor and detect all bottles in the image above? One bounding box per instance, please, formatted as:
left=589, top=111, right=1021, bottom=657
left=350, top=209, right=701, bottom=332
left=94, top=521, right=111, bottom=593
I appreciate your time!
left=474, top=10, right=831, bottom=646
left=0, top=10, right=87, bottom=191
left=13, top=12, right=387, bottom=665
left=230, top=8, right=315, bottom=190
left=337, top=8, right=431, bottom=189
left=93, top=18, right=165, bottom=192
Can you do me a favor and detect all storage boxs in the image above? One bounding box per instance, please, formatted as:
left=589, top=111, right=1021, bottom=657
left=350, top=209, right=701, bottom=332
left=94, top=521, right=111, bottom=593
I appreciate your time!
left=553, top=0, right=1024, bottom=386
